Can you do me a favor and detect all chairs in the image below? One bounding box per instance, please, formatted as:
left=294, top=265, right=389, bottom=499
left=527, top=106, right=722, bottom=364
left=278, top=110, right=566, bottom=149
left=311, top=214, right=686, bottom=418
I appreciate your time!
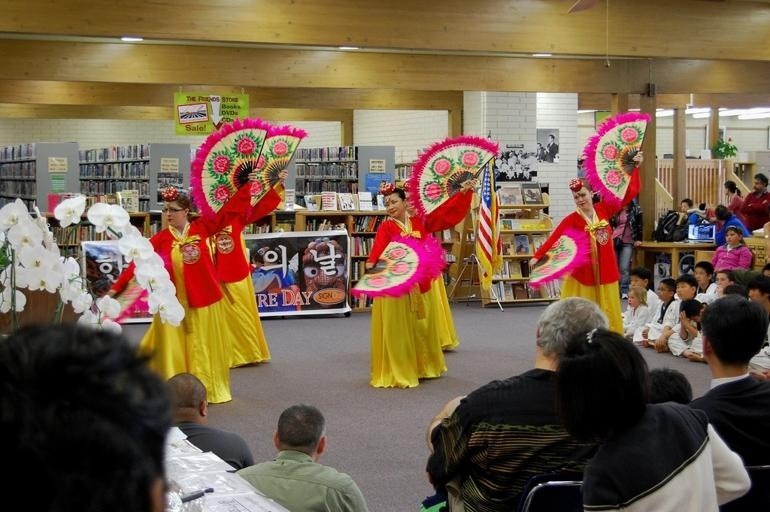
left=715, top=464, right=770, bottom=511
left=521, top=479, right=586, bottom=511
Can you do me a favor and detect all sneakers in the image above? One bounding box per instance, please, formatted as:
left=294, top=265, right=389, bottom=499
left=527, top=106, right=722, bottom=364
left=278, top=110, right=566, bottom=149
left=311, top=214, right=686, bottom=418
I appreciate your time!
left=622, top=292, right=628, bottom=300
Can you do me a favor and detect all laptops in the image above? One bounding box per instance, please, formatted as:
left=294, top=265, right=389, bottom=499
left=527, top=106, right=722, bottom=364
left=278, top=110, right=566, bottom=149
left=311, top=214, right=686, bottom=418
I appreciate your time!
left=687, top=223, right=716, bottom=243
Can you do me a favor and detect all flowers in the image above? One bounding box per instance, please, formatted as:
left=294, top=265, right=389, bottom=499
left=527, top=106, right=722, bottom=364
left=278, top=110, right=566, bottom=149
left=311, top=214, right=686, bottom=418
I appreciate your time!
left=0, top=191, right=186, bottom=340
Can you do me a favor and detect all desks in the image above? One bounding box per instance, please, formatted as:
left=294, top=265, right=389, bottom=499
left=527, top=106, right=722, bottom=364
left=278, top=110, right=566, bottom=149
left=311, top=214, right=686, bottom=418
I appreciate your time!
left=634, top=241, right=716, bottom=279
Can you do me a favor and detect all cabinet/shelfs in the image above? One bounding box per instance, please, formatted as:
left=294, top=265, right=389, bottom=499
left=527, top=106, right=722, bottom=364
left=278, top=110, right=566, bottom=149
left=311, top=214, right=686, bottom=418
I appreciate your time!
left=443, top=211, right=481, bottom=302
left=285, top=145, right=396, bottom=199
left=148, top=206, right=277, bottom=250
left=392, top=161, right=415, bottom=186
left=32, top=211, right=149, bottom=259
left=291, top=209, right=480, bottom=312
left=79, top=143, right=191, bottom=213
left=0, top=141, right=84, bottom=213
left=474, top=181, right=561, bottom=306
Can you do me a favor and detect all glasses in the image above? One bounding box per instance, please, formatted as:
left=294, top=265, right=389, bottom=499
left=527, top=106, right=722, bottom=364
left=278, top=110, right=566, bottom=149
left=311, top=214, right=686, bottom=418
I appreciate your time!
left=161, top=206, right=184, bottom=213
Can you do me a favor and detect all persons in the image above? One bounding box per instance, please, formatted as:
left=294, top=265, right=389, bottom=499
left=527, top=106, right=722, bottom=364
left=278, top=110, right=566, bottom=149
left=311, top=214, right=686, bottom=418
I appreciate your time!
left=536, top=134, right=560, bottom=162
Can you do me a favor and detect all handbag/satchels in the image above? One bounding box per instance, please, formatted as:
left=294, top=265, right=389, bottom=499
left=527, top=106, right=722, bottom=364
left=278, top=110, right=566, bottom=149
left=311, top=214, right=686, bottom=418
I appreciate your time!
left=613, top=236, right=622, bottom=249
left=654, top=210, right=688, bottom=242
